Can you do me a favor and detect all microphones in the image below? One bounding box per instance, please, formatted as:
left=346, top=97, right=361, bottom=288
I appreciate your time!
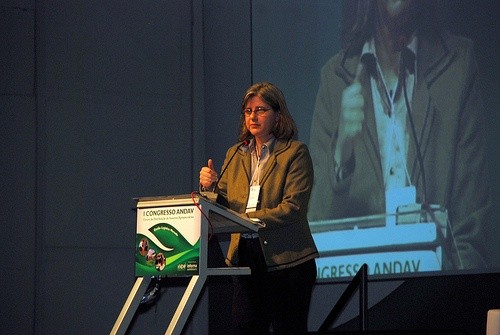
left=398, top=47, right=432, bottom=209
left=213, top=138, right=250, bottom=192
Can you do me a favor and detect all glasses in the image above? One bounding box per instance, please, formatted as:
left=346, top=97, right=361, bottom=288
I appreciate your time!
left=242, top=106, right=273, bottom=115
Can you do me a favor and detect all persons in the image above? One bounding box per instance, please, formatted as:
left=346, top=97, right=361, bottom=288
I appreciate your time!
left=199, top=82, right=319, bottom=335
left=306, top=0, right=499, bottom=271
